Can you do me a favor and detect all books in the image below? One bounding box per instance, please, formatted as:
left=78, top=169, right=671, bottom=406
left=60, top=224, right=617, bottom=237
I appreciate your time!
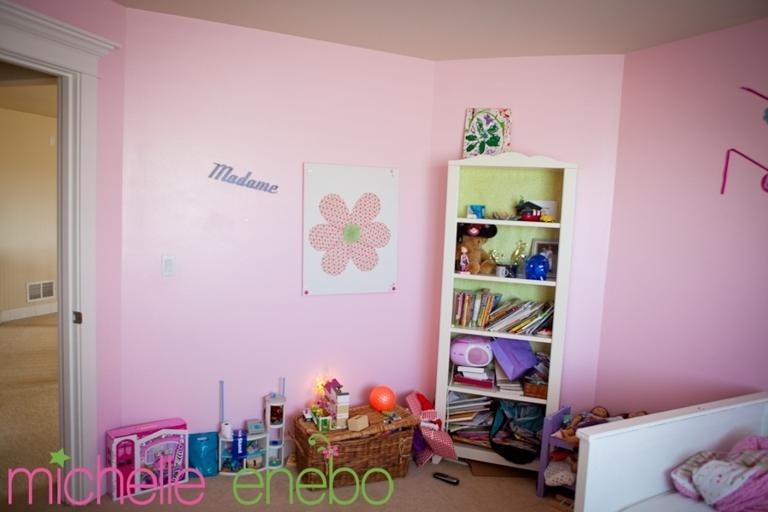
left=445, top=289, right=555, bottom=449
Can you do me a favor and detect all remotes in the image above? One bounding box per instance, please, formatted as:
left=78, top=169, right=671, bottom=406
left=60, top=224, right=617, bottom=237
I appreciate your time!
left=433, top=472, right=459, bottom=484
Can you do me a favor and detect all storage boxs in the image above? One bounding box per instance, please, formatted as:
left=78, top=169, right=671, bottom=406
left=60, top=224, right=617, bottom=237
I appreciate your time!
left=106, top=418, right=190, bottom=502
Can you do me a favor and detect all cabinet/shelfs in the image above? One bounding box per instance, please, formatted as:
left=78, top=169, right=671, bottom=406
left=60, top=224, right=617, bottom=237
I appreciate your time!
left=218, top=432, right=270, bottom=476
left=266, top=402, right=285, bottom=469
left=432, top=151, right=579, bottom=473
left=536, top=406, right=578, bottom=497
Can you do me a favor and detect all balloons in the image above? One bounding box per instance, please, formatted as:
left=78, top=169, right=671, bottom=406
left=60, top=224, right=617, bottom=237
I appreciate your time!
left=369, top=385, right=396, bottom=411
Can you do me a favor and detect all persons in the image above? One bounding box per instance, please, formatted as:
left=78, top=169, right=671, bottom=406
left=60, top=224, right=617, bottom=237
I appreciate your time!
left=544, top=402, right=649, bottom=490
left=456, top=223, right=497, bottom=274
left=540, top=246, right=553, bottom=272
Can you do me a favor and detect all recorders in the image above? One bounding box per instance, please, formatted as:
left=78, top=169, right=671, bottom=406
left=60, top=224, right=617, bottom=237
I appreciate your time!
left=450, top=335, right=494, bottom=366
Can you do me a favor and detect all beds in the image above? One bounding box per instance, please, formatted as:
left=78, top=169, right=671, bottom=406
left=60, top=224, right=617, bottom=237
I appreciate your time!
left=573, top=392, right=768, bottom=512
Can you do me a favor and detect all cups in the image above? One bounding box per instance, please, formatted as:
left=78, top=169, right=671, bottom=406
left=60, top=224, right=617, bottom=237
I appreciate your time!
left=496, top=267, right=508, bottom=278
